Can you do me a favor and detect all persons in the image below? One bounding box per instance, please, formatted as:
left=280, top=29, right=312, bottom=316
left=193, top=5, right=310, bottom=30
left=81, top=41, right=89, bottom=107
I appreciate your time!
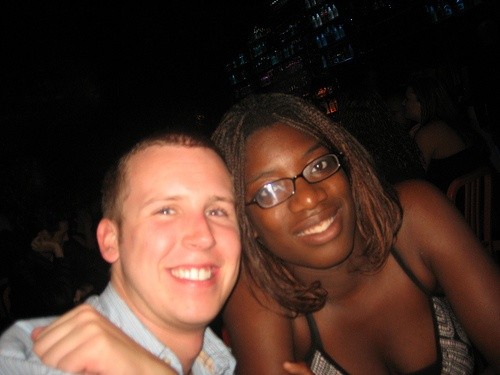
left=338, top=77, right=482, bottom=212
left=0, top=128, right=241, bottom=375
left=210, top=91, right=500, bottom=375
left=0, top=153, right=111, bottom=328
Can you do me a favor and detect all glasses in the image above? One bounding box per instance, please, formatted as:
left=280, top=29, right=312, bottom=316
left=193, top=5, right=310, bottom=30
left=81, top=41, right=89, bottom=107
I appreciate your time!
left=244, top=151, right=346, bottom=208
